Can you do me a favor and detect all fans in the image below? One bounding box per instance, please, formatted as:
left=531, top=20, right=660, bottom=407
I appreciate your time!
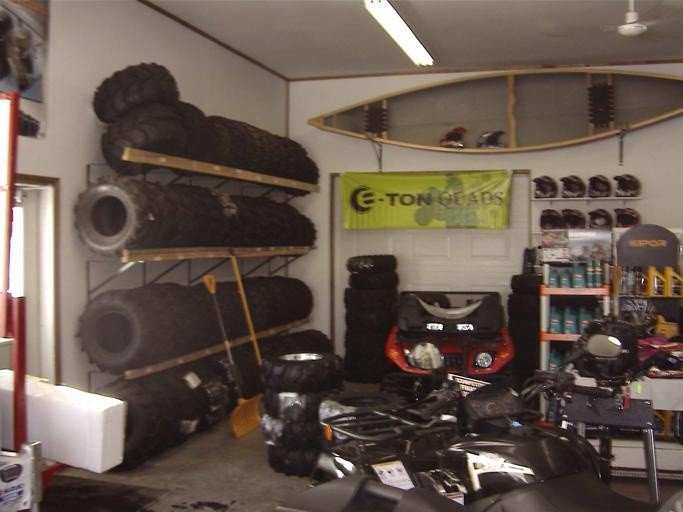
left=539, top=1, right=683, bottom=40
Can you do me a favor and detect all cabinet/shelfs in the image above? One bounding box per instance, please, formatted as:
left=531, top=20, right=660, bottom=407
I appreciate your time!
left=537, top=261, right=612, bottom=423
left=85, top=147, right=320, bottom=392
left=531, top=193, right=643, bottom=233
left=577, top=223, right=683, bottom=482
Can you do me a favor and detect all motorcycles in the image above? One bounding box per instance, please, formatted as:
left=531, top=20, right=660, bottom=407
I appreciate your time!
left=312, top=334, right=623, bottom=507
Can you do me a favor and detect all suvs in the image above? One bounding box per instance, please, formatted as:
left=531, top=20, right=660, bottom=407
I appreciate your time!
left=378, top=288, right=517, bottom=397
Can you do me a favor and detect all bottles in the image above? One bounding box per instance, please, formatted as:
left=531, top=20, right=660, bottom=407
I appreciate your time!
left=618, top=265, right=630, bottom=297
left=619, top=370, right=631, bottom=413
left=613, top=391, right=624, bottom=416
left=585, top=258, right=595, bottom=287
left=593, top=257, right=603, bottom=287
left=631, top=264, right=645, bottom=295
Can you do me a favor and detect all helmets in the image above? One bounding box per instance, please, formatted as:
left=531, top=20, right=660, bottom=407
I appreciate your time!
left=540, top=207, right=640, bottom=229
left=533, top=174, right=641, bottom=197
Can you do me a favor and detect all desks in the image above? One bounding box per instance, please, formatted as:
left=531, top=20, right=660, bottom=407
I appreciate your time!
left=560, top=397, right=661, bottom=505
